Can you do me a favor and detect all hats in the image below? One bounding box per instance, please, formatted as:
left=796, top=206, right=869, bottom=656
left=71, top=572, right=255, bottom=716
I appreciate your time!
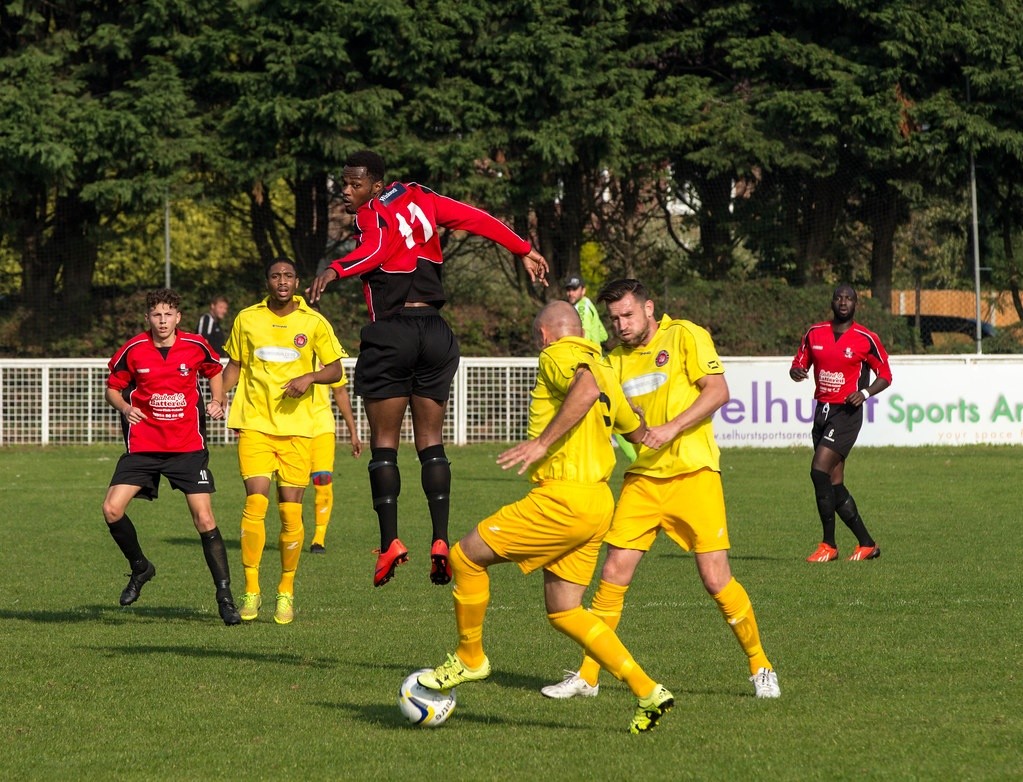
left=563, top=275, right=584, bottom=288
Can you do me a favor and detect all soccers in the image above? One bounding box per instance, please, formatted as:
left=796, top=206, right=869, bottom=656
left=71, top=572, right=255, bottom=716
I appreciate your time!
left=398, top=667, right=456, bottom=727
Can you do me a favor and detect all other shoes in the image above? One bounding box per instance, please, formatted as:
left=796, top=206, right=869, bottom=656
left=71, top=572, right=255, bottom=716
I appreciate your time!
left=310, top=543, right=325, bottom=553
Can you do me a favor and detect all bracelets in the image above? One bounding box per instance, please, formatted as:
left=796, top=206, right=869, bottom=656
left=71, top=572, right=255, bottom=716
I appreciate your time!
left=211, top=400, right=220, bottom=405
left=860, top=389, right=869, bottom=399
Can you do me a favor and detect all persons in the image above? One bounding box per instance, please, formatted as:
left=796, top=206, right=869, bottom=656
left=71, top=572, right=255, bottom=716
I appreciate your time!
left=417, top=300, right=676, bottom=734
left=221, top=257, right=350, bottom=624
left=540, top=279, right=781, bottom=697
left=304, top=151, right=549, bottom=587
left=565, top=274, right=637, bottom=463
left=196, top=293, right=229, bottom=399
left=103, top=290, right=242, bottom=627
left=789, top=285, right=893, bottom=563
left=305, top=296, right=362, bottom=555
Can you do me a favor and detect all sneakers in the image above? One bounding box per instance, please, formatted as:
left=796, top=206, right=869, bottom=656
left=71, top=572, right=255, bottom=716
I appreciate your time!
left=240, top=593, right=262, bottom=620
left=541, top=669, right=598, bottom=698
left=626, top=684, right=674, bottom=734
left=216, top=593, right=239, bottom=626
left=805, top=542, right=838, bottom=562
left=846, top=542, right=880, bottom=560
left=372, top=539, right=408, bottom=587
left=749, top=666, right=780, bottom=698
left=428, top=539, right=452, bottom=585
left=417, top=651, right=492, bottom=691
left=273, top=592, right=294, bottom=624
left=120, top=560, right=156, bottom=606
